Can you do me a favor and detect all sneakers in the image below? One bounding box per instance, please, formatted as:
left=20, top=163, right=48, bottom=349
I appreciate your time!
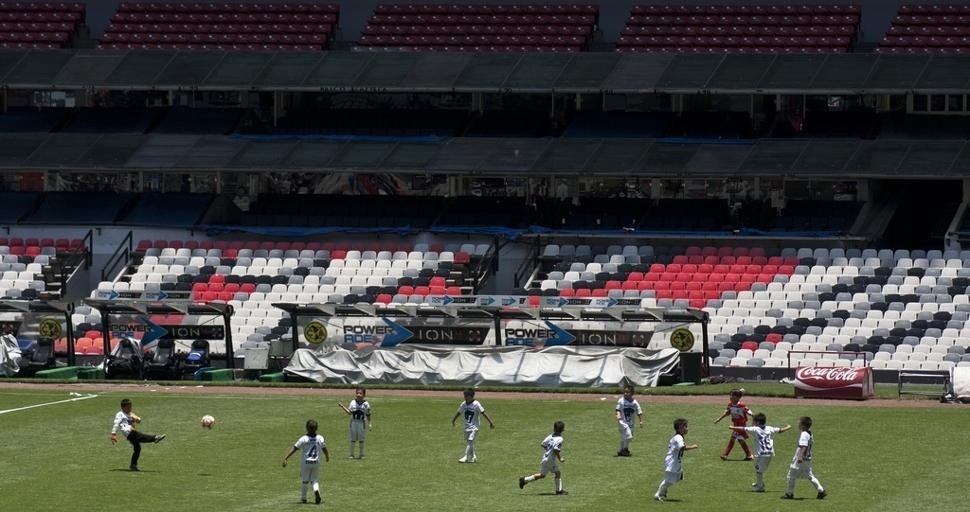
left=154, top=434, right=165, bottom=443
left=780, top=493, right=793, bottom=499
left=744, top=456, right=753, bottom=461
left=556, top=490, right=568, bottom=495
left=720, top=456, right=727, bottom=461
left=130, top=465, right=142, bottom=471
left=315, top=491, right=321, bottom=504
left=468, top=454, right=476, bottom=463
left=458, top=455, right=467, bottom=463
left=520, top=476, right=525, bottom=489
left=816, top=490, right=827, bottom=499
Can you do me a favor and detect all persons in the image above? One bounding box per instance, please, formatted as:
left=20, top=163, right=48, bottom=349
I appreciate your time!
left=336, top=386, right=373, bottom=460
left=726, top=412, right=792, bottom=493
left=714, top=387, right=754, bottom=461
left=780, top=416, right=829, bottom=501
left=281, top=419, right=329, bottom=505
left=654, top=418, right=697, bottom=502
left=109, top=398, right=165, bottom=473
left=451, top=386, right=495, bottom=463
left=519, top=419, right=571, bottom=497
left=614, top=384, right=645, bottom=458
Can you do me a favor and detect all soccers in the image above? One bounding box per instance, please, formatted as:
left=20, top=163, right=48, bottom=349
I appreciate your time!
left=200, top=415, right=215, bottom=429
